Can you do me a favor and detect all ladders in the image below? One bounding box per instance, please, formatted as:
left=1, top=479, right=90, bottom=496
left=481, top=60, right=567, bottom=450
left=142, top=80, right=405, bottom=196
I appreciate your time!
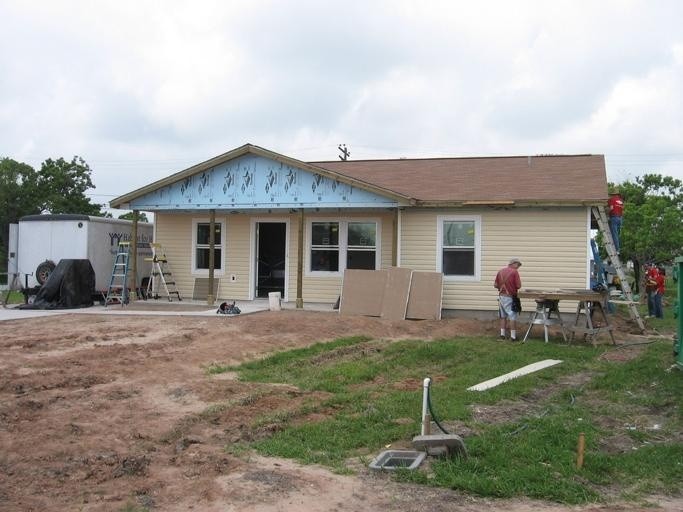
left=104, top=241, right=140, bottom=307
left=591, top=206, right=648, bottom=337
left=144, top=242, right=183, bottom=302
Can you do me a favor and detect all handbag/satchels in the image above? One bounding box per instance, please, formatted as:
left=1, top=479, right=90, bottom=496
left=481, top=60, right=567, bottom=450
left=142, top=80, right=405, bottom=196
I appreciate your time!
left=512, top=296, right=522, bottom=313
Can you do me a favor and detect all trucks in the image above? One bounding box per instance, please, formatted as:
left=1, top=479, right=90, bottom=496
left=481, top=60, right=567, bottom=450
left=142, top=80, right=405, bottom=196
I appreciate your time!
left=7, top=214, right=154, bottom=299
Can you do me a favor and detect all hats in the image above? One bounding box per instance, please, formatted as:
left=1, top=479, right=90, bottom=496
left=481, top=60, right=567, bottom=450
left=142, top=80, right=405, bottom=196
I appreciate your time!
left=508, top=258, right=522, bottom=265
left=609, top=190, right=621, bottom=195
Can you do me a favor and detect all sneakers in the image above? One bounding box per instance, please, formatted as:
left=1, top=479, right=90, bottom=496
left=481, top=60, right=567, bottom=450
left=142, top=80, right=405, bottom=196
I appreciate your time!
left=644, top=315, right=656, bottom=319
left=496, top=336, right=505, bottom=340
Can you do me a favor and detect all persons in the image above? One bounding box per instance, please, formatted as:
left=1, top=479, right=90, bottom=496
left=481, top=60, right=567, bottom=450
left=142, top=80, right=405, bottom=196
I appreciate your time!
left=642, top=262, right=666, bottom=320
left=493, top=257, right=522, bottom=343
left=604, top=187, right=625, bottom=256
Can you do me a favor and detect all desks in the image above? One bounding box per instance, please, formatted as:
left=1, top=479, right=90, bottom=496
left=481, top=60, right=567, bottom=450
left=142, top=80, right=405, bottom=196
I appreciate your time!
left=518, top=289, right=617, bottom=347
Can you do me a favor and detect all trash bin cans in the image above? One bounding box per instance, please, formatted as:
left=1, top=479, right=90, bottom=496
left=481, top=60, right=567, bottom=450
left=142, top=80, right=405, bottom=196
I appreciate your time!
left=268, top=292, right=282, bottom=312
